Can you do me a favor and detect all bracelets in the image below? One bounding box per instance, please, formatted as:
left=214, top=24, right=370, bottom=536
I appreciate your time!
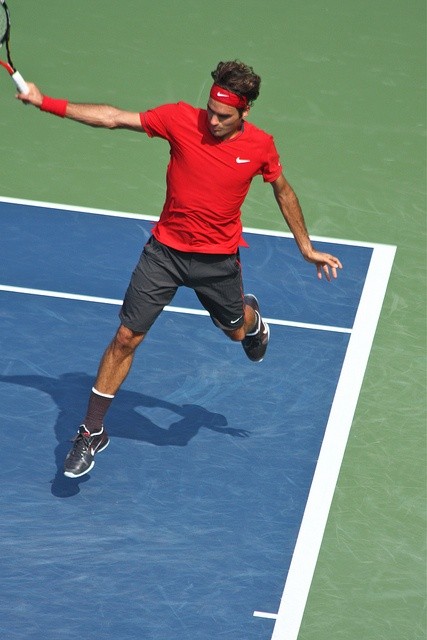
left=39, top=95, right=68, bottom=120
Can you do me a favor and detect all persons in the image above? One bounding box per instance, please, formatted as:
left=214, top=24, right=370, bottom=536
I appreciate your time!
left=14, top=61, right=342, bottom=481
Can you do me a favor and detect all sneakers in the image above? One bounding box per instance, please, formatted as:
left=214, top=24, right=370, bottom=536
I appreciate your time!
left=240, top=293, right=270, bottom=363
left=62, top=423, right=110, bottom=478
